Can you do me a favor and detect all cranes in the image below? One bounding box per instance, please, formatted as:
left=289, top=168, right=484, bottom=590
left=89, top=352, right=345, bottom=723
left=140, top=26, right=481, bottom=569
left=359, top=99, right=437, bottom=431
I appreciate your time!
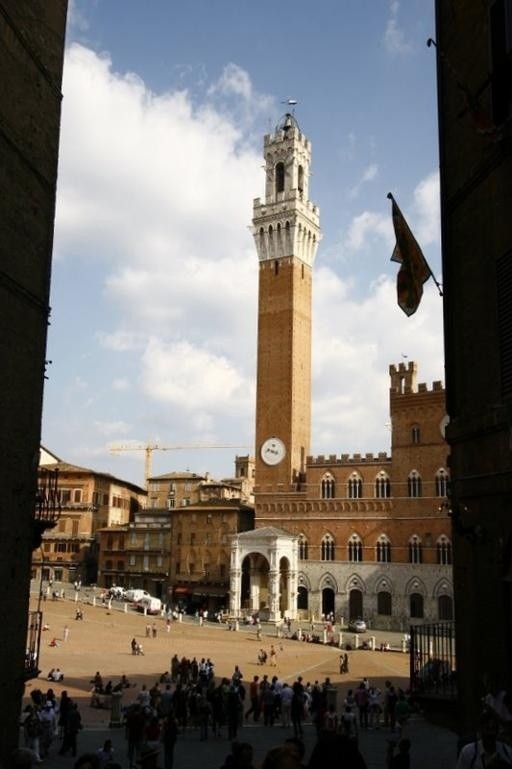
left=108, top=439, right=256, bottom=492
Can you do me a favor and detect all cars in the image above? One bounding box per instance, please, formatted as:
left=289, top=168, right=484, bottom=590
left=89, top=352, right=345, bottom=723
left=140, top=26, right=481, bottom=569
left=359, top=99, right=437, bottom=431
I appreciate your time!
left=348, top=619, right=367, bottom=633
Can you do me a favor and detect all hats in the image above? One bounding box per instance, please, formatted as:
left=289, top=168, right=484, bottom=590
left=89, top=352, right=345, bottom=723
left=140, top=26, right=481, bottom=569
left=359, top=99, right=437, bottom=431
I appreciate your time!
left=43, top=700, right=53, bottom=707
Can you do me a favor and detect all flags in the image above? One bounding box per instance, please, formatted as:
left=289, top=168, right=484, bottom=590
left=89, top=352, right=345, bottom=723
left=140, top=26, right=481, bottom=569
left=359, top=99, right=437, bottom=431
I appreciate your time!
left=391, top=197, right=430, bottom=316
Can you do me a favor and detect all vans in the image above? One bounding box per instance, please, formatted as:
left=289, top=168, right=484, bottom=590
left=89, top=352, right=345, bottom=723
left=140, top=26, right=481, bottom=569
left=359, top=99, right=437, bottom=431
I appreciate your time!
left=110, top=584, right=163, bottom=615
